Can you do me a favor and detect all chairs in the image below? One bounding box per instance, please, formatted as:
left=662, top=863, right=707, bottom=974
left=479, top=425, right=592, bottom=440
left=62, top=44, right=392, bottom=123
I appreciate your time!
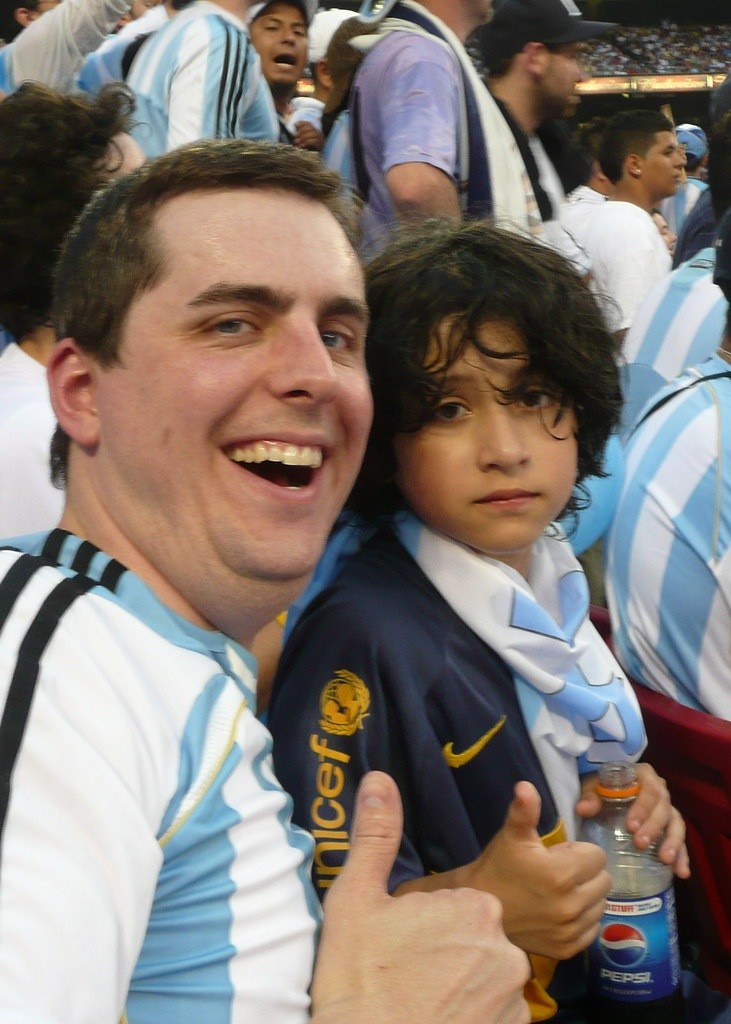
left=585, top=602, right=731, bottom=997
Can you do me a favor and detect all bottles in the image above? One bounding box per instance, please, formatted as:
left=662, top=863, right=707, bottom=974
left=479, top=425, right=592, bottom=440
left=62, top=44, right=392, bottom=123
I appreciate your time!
left=579, top=759, right=684, bottom=1008
left=284, top=77, right=324, bottom=147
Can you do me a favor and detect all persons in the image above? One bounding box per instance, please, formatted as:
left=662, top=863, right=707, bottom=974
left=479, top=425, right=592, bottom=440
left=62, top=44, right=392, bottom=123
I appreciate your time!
left=0, top=138, right=530, bottom=1023
left=269, top=229, right=731, bottom=1023
left=0, top=0, right=731, bottom=720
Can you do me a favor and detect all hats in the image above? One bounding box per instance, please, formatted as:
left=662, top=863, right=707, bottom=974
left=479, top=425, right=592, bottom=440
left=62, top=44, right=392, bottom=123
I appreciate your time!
left=490, top=0, right=619, bottom=44
left=676, top=123, right=708, bottom=167
left=307, top=8, right=360, bottom=63
left=246, top=0, right=309, bottom=25
